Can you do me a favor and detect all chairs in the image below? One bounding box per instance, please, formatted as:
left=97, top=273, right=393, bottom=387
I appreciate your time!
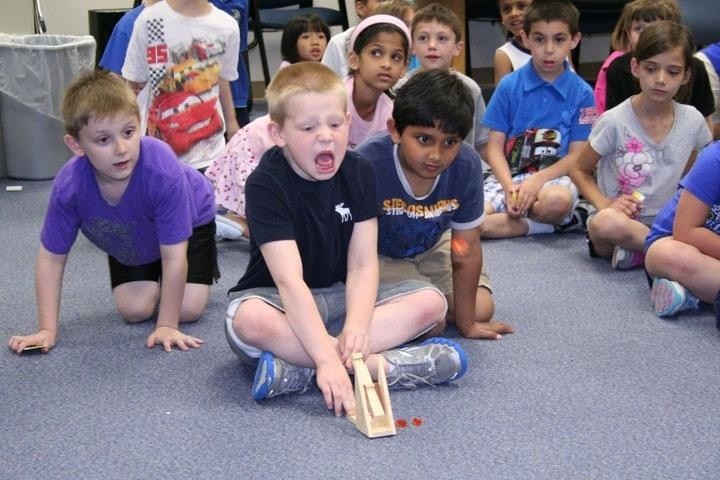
left=233, top=0, right=351, bottom=93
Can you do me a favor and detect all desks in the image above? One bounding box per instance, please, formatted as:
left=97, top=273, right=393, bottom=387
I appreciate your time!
left=462, top=0, right=631, bottom=105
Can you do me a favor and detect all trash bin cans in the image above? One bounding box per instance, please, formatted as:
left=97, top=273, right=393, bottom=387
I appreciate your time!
left=0, top=34, right=97, bottom=181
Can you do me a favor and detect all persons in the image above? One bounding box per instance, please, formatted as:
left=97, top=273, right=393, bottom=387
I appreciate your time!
left=98, top=0, right=159, bottom=78
left=7, top=68, right=221, bottom=352
left=351, top=66, right=515, bottom=339
left=372, top=0, right=419, bottom=70
left=204, top=13, right=413, bottom=237
left=277, top=14, right=331, bottom=72
left=391, top=2, right=490, bottom=164
left=479, top=0, right=720, bottom=331
left=96, top=0, right=241, bottom=174
left=224, top=59, right=469, bottom=419
left=319, top=0, right=385, bottom=76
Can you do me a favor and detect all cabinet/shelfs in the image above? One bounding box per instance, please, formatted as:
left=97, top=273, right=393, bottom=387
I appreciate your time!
left=87, top=7, right=132, bottom=67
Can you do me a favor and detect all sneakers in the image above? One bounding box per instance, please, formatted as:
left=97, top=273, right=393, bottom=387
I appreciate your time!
left=555, top=201, right=593, bottom=233
left=212, top=214, right=245, bottom=242
left=649, top=275, right=698, bottom=318
left=250, top=349, right=317, bottom=401
left=380, top=335, right=469, bottom=387
left=610, top=244, right=645, bottom=273
